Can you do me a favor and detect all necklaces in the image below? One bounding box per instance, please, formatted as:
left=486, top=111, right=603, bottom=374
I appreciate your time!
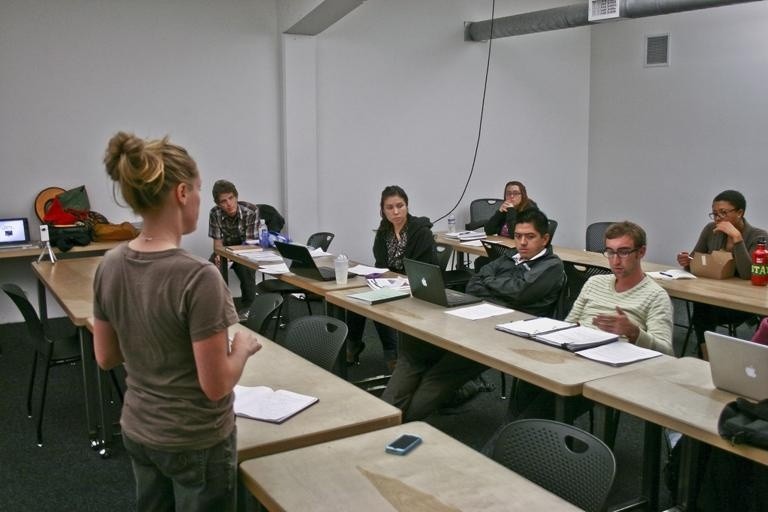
left=136, top=231, right=178, bottom=246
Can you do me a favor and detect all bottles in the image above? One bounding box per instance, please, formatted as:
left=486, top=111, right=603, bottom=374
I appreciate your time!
left=447, top=210, right=458, bottom=232
left=258, top=219, right=269, bottom=247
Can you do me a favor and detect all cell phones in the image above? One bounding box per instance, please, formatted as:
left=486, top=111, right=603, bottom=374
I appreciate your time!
left=385, top=433, right=422, bottom=455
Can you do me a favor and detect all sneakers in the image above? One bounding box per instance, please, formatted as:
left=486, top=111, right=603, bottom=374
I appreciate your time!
left=347, top=349, right=354, bottom=367
left=237, top=308, right=250, bottom=324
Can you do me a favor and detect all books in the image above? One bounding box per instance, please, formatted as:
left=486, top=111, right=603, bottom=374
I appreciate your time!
left=496, top=316, right=620, bottom=352
left=230, top=384, right=320, bottom=425
left=347, top=285, right=411, bottom=305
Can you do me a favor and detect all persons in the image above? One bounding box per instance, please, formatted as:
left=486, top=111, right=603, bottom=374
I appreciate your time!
left=680, top=191, right=768, bottom=357
left=476, top=222, right=676, bottom=461
left=380, top=210, right=567, bottom=426
left=92, top=130, right=263, bottom=512
left=345, top=187, right=439, bottom=371
left=204, top=179, right=260, bottom=322
left=484, top=182, right=540, bottom=235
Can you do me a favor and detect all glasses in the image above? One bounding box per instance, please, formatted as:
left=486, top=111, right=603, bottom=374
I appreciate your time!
left=601, top=248, right=639, bottom=258
left=708, top=207, right=736, bottom=220
left=506, top=192, right=522, bottom=197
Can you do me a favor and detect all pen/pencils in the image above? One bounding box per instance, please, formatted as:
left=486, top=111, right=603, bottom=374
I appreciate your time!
left=678, top=252, right=694, bottom=262
left=659, top=271, right=673, bottom=278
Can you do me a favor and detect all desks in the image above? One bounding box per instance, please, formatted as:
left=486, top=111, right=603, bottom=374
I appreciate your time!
left=583, top=355, right=768, bottom=512
left=31, top=256, right=132, bottom=449
left=237, top=417, right=582, bottom=511
left=324, top=280, right=676, bottom=512
left=431, top=230, right=768, bottom=317
left=0, top=238, right=131, bottom=325
left=84, top=317, right=401, bottom=463
left=213, top=244, right=408, bottom=386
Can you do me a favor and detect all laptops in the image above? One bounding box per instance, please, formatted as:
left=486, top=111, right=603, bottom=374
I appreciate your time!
left=274, top=239, right=359, bottom=282
left=402, top=257, right=483, bottom=308
left=704, top=329, right=768, bottom=402
left=0, top=217, right=33, bottom=248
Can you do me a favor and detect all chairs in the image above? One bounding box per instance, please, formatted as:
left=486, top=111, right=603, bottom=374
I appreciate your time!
left=546, top=219, right=558, bottom=244
left=283, top=315, right=349, bottom=373
left=250, top=204, right=286, bottom=281
left=492, top=418, right=617, bottom=511
left=562, top=259, right=614, bottom=307
left=433, top=242, right=453, bottom=273
left=585, top=221, right=618, bottom=252
left=0, top=283, right=124, bottom=447
left=246, top=292, right=284, bottom=334
left=469, top=198, right=505, bottom=231
left=480, top=238, right=512, bottom=260
left=256, top=231, right=336, bottom=341
